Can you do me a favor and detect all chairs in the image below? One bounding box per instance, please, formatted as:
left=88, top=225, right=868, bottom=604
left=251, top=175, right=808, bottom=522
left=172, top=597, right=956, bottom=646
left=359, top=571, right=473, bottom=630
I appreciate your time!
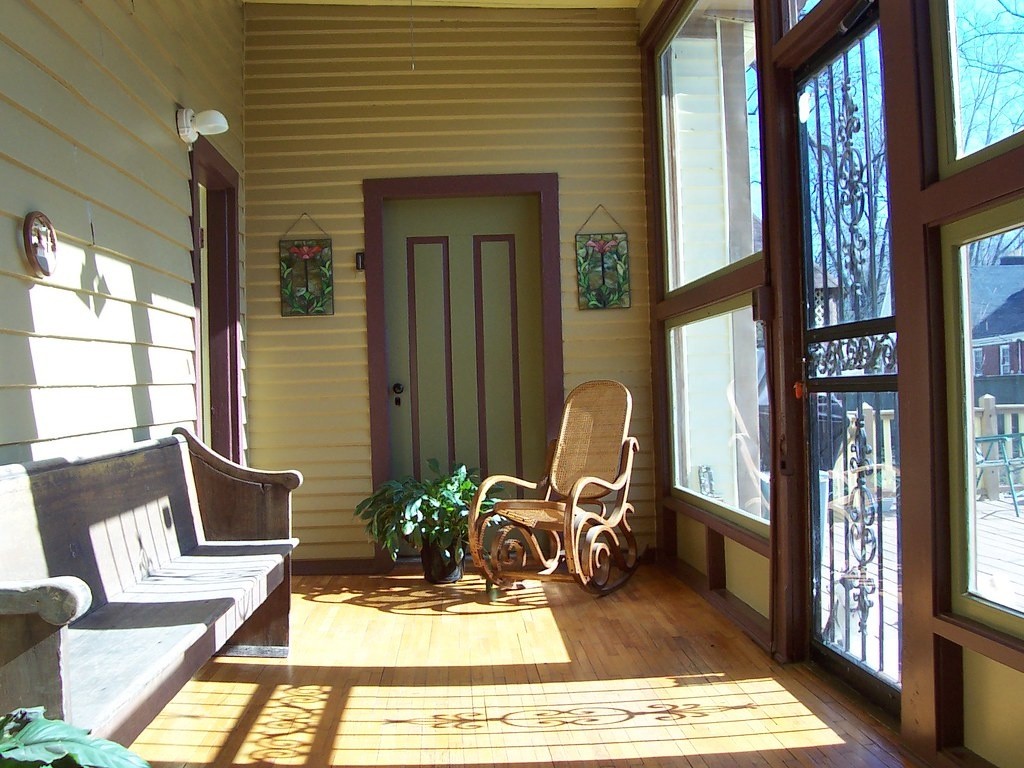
left=467, top=379, right=647, bottom=595
left=726, top=381, right=901, bottom=585
left=974, top=433, right=1024, bottom=517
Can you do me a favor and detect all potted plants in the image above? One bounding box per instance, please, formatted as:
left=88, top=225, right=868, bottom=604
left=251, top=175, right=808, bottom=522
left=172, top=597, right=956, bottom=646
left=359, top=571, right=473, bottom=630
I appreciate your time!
left=352, top=458, right=503, bottom=584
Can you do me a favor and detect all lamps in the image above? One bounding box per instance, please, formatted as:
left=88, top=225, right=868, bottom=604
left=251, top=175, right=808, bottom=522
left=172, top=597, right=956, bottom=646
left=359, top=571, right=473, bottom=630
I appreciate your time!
left=177, top=108, right=230, bottom=143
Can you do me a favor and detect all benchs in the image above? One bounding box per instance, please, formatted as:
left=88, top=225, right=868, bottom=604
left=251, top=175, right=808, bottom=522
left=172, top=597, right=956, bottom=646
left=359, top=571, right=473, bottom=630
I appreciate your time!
left=0, top=426, right=303, bottom=748
